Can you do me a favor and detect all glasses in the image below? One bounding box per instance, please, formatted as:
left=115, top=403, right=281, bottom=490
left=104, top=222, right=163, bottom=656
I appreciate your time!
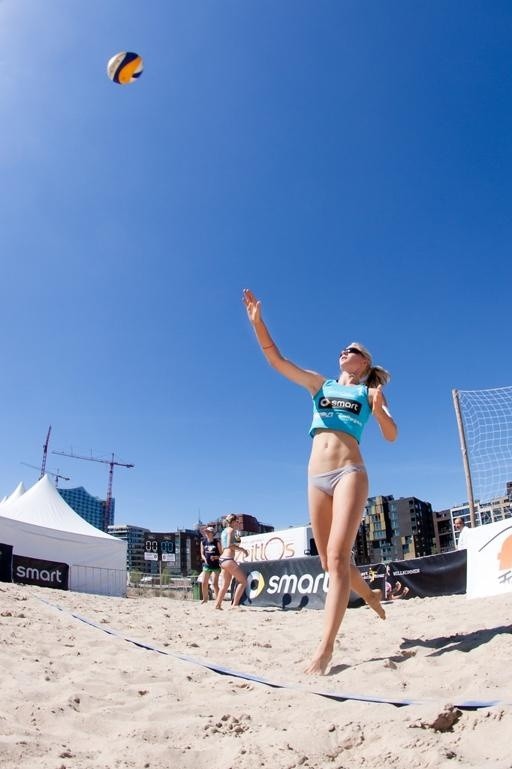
left=339, top=347, right=364, bottom=358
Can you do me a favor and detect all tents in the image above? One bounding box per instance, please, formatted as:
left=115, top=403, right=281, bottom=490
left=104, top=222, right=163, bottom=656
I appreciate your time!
left=0, top=474, right=129, bottom=597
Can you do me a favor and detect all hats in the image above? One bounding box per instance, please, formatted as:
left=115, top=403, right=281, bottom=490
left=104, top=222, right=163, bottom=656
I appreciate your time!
left=205, top=527, right=216, bottom=533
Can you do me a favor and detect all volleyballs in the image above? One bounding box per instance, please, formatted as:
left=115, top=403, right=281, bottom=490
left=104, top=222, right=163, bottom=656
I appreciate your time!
left=107, top=52, right=143, bottom=85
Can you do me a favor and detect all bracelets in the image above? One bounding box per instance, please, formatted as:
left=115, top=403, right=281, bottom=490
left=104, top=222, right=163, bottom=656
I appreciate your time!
left=261, top=343, right=275, bottom=350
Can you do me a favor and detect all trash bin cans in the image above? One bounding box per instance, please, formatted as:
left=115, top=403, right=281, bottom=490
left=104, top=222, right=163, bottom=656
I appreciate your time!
left=190, top=581, right=211, bottom=602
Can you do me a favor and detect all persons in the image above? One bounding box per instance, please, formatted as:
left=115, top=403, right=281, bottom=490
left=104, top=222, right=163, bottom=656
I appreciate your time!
left=454, top=517, right=469, bottom=551
left=198, top=527, right=222, bottom=604
left=214, top=512, right=248, bottom=612
left=384, top=563, right=410, bottom=601
left=241, top=288, right=397, bottom=676
